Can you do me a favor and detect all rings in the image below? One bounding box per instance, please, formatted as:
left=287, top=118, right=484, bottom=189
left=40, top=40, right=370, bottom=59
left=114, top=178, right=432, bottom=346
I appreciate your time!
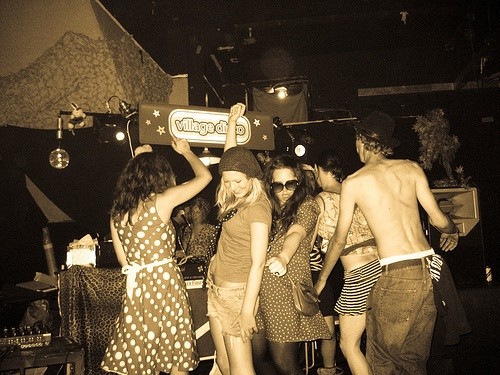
left=275, top=272, right=280, bottom=276
left=177, top=138, right=180, bottom=140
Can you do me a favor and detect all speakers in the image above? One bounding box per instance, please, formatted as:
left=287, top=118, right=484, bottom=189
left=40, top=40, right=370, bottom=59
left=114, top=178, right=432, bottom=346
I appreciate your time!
left=427, top=187, right=487, bottom=291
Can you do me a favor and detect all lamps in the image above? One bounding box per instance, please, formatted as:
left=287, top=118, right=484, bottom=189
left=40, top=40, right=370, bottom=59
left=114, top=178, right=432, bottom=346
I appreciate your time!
left=96, top=126, right=128, bottom=145
left=49, top=118, right=69, bottom=169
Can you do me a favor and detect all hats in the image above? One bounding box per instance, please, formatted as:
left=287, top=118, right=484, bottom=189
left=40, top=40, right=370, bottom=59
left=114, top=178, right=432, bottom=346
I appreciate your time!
left=218, top=146, right=264, bottom=180
left=347, top=112, right=398, bottom=145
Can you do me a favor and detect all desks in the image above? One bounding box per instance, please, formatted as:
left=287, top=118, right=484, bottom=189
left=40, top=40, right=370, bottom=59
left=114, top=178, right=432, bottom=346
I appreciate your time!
left=0, top=336, right=85, bottom=375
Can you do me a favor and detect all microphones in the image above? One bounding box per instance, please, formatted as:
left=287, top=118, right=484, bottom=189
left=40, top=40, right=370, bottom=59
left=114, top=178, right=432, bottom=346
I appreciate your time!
left=179, top=210, right=190, bottom=228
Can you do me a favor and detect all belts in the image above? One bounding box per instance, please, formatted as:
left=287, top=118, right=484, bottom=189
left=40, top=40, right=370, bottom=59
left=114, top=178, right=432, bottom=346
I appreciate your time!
left=381, top=254, right=433, bottom=272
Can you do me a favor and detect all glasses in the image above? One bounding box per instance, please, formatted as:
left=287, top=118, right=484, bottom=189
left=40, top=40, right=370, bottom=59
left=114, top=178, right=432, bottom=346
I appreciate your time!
left=271, top=180, right=299, bottom=193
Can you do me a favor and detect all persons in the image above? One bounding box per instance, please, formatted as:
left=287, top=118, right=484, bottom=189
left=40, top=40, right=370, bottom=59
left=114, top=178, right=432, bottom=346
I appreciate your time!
left=99, top=136, right=212, bottom=375
left=206, top=103, right=273, bottom=375
left=316, top=152, right=382, bottom=375
left=314, top=112, right=459, bottom=375
left=171, top=202, right=192, bottom=265
left=302, top=163, right=337, bottom=375
left=251, top=153, right=332, bottom=375
left=181, top=198, right=217, bottom=264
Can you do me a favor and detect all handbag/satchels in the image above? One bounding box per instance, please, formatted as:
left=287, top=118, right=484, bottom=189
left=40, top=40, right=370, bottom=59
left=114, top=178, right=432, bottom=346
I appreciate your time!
left=293, top=282, right=320, bottom=317
